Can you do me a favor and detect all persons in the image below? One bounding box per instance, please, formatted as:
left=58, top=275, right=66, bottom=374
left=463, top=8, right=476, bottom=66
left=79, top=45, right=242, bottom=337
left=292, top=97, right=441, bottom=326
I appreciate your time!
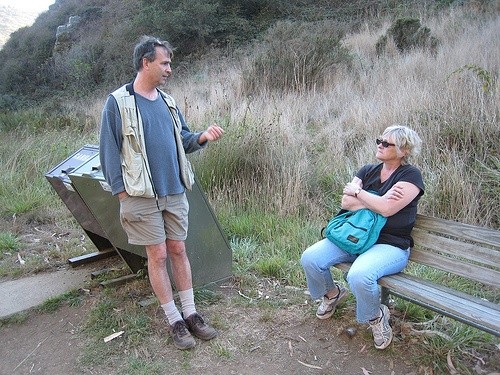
left=100, top=37, right=225, bottom=351
left=301, top=126, right=425, bottom=350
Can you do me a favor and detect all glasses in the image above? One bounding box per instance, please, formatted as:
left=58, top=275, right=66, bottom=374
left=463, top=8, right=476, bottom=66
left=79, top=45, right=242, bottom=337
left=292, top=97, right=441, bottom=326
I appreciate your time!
left=377, top=139, right=395, bottom=148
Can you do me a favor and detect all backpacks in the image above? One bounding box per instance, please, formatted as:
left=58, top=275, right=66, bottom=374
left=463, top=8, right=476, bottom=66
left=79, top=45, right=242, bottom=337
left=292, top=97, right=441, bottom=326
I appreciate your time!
left=324, top=190, right=388, bottom=256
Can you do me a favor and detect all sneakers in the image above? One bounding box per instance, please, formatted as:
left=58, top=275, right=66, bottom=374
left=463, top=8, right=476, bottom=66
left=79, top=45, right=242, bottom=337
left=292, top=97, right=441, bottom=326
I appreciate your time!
left=167, top=319, right=196, bottom=349
left=367, top=304, right=392, bottom=350
left=315, top=284, right=347, bottom=320
left=181, top=312, right=217, bottom=340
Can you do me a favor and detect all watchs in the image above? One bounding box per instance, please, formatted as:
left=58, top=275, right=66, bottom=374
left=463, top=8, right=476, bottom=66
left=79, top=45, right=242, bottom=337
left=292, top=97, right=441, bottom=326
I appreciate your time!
left=355, top=188, right=363, bottom=197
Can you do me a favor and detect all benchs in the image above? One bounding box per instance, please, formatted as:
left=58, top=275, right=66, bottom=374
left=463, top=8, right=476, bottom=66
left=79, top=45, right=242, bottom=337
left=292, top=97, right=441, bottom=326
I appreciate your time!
left=332, top=211, right=500, bottom=338
